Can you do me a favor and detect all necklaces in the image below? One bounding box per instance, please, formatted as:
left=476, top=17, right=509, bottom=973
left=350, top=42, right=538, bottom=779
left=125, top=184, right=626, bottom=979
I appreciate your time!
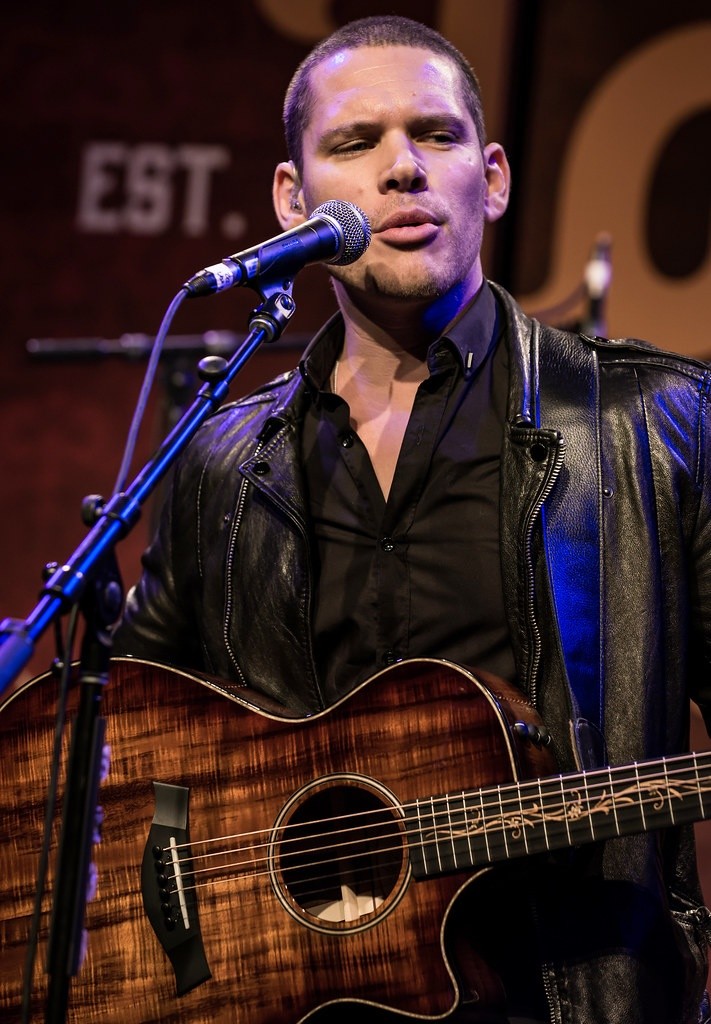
left=334, top=360, right=339, bottom=394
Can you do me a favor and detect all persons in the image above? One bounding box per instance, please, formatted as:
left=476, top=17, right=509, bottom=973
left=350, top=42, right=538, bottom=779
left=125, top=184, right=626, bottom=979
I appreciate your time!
left=109, top=17, right=711, bottom=1024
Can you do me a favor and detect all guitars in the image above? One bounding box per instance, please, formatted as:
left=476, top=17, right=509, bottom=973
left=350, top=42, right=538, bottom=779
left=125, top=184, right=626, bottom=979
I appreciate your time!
left=2, top=654, right=711, bottom=1022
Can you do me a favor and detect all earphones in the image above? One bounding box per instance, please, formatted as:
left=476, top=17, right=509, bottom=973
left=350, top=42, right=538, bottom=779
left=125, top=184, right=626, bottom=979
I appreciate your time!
left=289, top=191, right=304, bottom=213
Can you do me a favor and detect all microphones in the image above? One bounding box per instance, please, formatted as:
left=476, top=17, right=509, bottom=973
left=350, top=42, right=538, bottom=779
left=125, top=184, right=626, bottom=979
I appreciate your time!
left=585, top=233, right=613, bottom=339
left=183, top=200, right=371, bottom=300
